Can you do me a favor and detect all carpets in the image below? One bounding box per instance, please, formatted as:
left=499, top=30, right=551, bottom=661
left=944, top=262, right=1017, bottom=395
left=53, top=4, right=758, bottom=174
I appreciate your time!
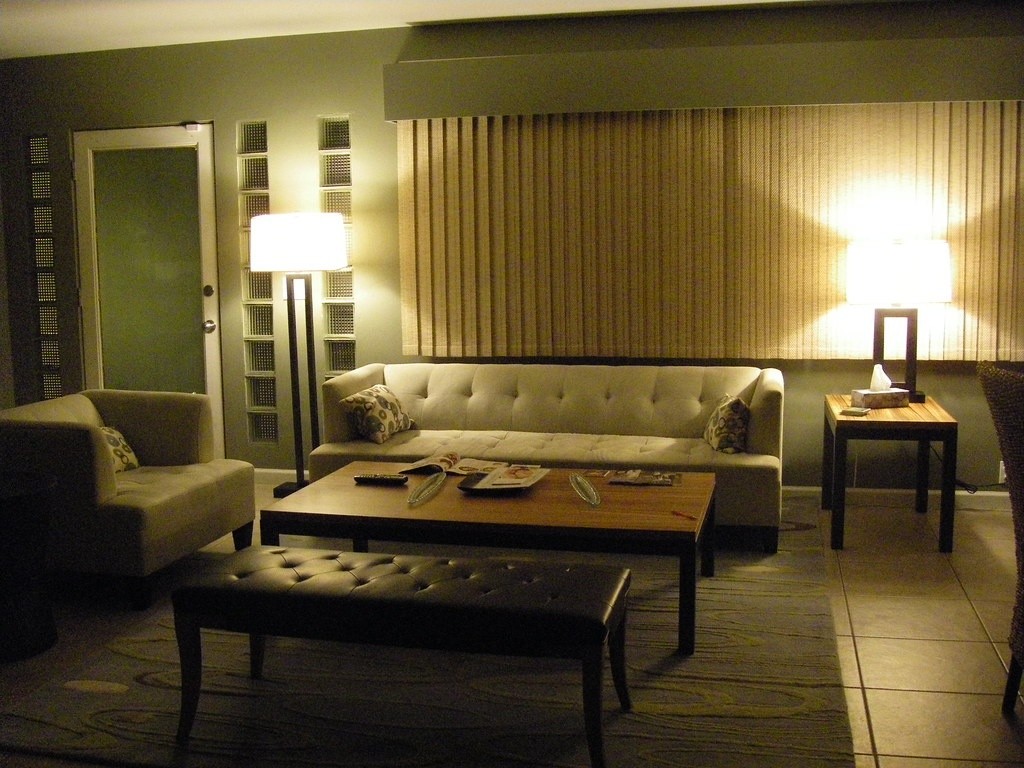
left=1, top=571, right=857, bottom=768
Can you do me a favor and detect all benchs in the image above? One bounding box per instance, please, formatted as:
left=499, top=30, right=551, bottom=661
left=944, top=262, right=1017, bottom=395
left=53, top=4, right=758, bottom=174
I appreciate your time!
left=172, top=546, right=632, bottom=768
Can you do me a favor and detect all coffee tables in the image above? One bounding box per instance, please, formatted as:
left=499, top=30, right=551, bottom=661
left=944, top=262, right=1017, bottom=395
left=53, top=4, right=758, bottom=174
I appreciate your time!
left=260, top=460, right=716, bottom=655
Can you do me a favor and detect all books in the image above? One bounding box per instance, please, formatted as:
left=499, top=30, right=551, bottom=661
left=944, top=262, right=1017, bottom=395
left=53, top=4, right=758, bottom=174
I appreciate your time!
left=399, top=451, right=509, bottom=475
left=492, top=463, right=542, bottom=487
left=608, top=468, right=684, bottom=486
left=581, top=469, right=610, bottom=478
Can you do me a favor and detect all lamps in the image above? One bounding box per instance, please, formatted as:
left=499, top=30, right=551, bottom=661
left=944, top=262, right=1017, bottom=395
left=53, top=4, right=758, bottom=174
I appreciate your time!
left=250, top=211, right=349, bottom=499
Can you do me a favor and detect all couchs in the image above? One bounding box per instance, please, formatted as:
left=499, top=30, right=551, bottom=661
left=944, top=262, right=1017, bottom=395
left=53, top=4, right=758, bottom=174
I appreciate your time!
left=309, top=362, right=784, bottom=554
left=0, top=389, right=255, bottom=610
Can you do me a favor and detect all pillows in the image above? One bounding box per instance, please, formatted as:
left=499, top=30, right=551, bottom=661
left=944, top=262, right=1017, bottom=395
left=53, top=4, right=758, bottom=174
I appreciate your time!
left=100, top=426, right=140, bottom=474
left=338, top=384, right=415, bottom=444
left=703, top=392, right=751, bottom=454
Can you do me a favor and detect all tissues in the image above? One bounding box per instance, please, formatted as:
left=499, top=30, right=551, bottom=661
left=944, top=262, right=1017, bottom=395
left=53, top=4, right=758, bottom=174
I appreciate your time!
left=851, top=364, right=911, bottom=407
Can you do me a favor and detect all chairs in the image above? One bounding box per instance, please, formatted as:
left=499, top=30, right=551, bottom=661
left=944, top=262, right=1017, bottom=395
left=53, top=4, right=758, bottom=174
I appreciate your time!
left=975, top=360, right=1024, bottom=718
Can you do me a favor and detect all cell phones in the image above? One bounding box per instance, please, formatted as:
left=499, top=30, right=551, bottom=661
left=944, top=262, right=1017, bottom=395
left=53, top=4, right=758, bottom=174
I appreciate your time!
left=843, top=407, right=871, bottom=415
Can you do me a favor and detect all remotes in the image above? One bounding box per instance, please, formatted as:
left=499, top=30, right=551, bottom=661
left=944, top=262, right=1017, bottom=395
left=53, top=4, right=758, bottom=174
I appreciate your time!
left=354, top=473, right=409, bottom=484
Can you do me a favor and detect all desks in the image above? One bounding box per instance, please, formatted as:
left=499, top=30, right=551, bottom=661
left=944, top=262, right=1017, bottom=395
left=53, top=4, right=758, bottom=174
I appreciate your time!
left=821, top=394, right=958, bottom=554
